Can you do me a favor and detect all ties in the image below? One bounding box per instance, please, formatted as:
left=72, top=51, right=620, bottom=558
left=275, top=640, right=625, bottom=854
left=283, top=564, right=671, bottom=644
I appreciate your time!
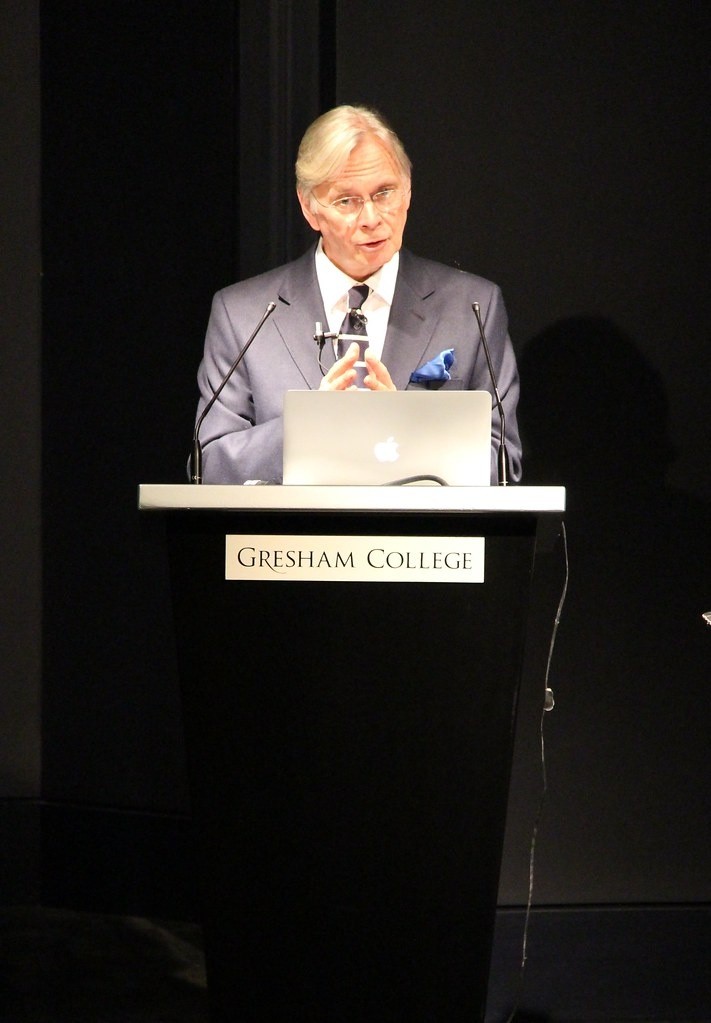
left=336, top=286, right=372, bottom=388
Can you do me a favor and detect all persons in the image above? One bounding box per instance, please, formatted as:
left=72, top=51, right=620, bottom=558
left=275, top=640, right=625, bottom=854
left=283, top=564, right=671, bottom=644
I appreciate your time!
left=195, top=102, right=522, bottom=494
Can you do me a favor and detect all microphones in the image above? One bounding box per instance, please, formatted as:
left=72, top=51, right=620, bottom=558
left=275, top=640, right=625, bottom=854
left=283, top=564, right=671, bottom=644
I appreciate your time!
left=193, top=301, right=278, bottom=486
left=471, top=301, right=509, bottom=486
left=349, top=308, right=368, bottom=332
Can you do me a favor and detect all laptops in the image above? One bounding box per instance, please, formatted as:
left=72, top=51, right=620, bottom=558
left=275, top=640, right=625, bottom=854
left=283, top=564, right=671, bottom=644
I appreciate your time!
left=283, top=388, right=492, bottom=486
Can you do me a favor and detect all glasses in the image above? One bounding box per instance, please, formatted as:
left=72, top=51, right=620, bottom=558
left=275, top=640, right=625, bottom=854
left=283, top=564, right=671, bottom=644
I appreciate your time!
left=311, top=187, right=412, bottom=222
